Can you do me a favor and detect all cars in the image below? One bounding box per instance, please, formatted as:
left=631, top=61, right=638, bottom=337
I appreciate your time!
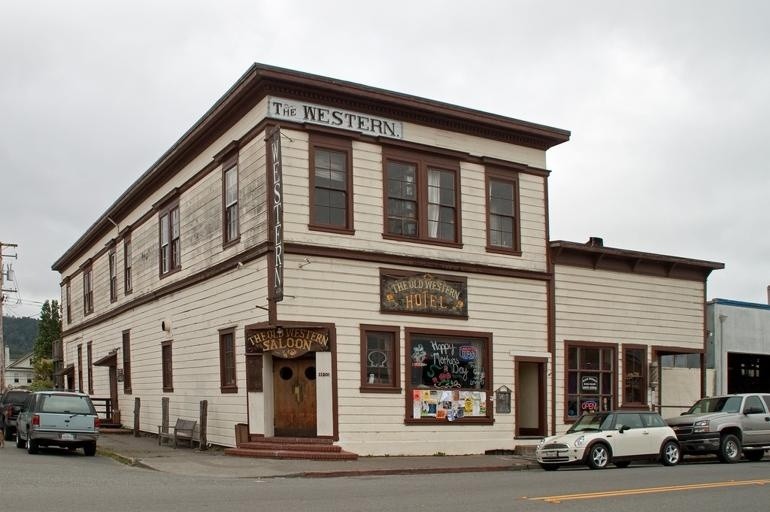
left=535, top=409, right=682, bottom=470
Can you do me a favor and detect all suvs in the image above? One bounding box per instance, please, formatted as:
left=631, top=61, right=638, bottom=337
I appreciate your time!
left=0, top=387, right=33, bottom=441
left=664, top=391, right=770, bottom=464
left=14, top=389, right=102, bottom=457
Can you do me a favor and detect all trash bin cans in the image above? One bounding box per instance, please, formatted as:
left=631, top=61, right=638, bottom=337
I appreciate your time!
left=235, top=424, right=248, bottom=445
left=113, top=409, right=119, bottom=424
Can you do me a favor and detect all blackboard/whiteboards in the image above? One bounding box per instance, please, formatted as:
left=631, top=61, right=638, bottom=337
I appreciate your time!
left=422, top=342, right=480, bottom=390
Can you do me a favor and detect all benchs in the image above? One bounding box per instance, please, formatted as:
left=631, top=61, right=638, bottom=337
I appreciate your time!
left=157, top=419, right=198, bottom=449
